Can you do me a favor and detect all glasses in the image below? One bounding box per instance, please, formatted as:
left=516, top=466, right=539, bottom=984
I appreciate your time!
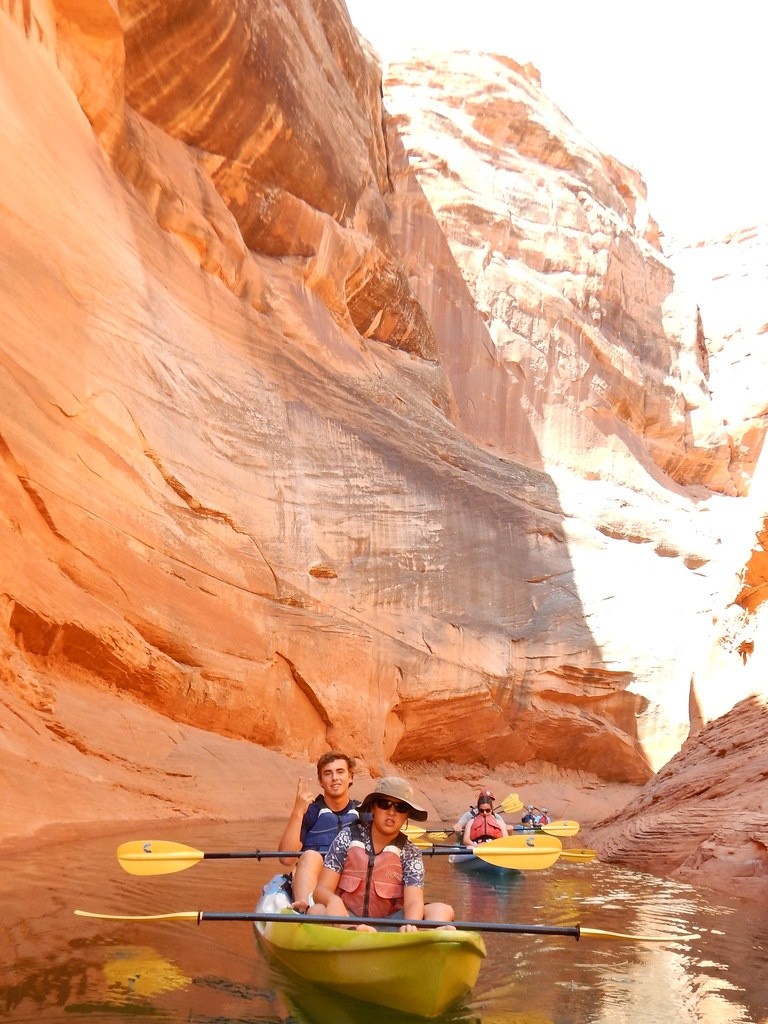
left=375, top=797, right=410, bottom=813
left=479, top=808, right=492, bottom=813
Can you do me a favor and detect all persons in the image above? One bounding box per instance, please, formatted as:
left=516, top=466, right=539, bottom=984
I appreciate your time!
left=278, top=751, right=363, bottom=914
left=521, top=805, right=536, bottom=826
left=533, top=808, right=551, bottom=827
left=309, top=777, right=456, bottom=935
left=454, top=790, right=514, bottom=848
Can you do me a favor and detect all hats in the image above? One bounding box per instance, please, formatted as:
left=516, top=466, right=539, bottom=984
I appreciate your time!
left=354, top=777, right=429, bottom=822
left=479, top=791, right=497, bottom=802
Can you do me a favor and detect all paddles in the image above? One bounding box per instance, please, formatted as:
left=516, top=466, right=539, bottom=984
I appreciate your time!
left=399, top=820, right=580, bottom=840
left=523, top=805, right=537, bottom=817
left=115, top=833, right=564, bottom=877
left=534, top=807, right=541, bottom=812
left=411, top=838, right=597, bottom=863
left=71, top=907, right=703, bottom=945
left=428, top=792, right=524, bottom=841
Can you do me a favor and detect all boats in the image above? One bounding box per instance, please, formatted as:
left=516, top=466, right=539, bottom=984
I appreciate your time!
left=448, top=838, right=493, bottom=864
left=253, top=873, right=487, bottom=1024
left=516, top=825, right=531, bottom=833
